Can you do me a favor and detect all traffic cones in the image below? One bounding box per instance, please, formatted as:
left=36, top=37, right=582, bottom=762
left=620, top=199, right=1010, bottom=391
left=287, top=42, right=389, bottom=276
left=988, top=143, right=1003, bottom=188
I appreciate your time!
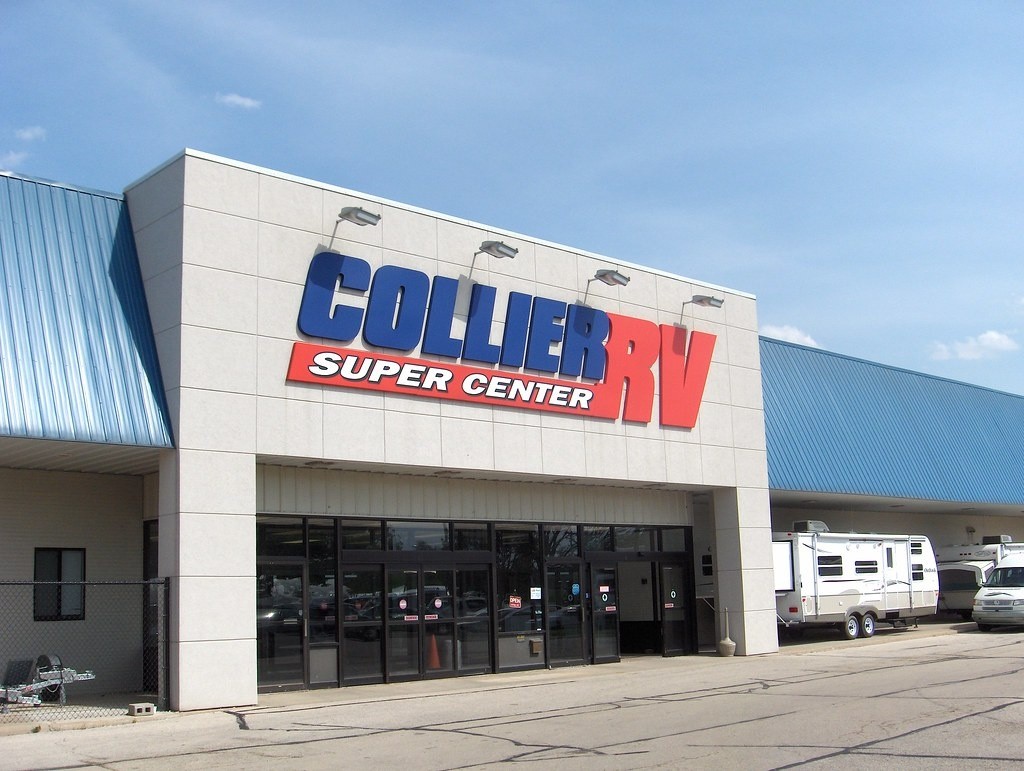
left=427, top=634, right=442, bottom=671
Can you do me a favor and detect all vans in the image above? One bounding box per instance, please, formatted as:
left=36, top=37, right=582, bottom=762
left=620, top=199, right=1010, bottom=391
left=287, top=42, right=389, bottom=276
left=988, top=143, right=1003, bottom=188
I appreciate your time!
left=971, top=542, right=1024, bottom=632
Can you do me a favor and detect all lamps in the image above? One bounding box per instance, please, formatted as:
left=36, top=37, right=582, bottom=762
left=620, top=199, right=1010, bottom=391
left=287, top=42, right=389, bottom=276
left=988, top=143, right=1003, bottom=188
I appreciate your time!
left=468, top=239, right=519, bottom=281
left=583, top=269, right=631, bottom=306
left=680, top=295, right=725, bottom=327
left=323, top=206, right=382, bottom=253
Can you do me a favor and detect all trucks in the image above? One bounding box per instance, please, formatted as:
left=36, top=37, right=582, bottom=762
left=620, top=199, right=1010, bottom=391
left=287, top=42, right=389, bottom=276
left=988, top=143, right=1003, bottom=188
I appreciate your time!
left=933, top=535, right=1024, bottom=622
left=774, top=520, right=941, bottom=642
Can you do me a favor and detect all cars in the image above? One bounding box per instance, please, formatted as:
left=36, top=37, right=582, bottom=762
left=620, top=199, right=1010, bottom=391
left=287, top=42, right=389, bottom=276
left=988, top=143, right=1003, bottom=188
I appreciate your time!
left=256, top=588, right=582, bottom=635
